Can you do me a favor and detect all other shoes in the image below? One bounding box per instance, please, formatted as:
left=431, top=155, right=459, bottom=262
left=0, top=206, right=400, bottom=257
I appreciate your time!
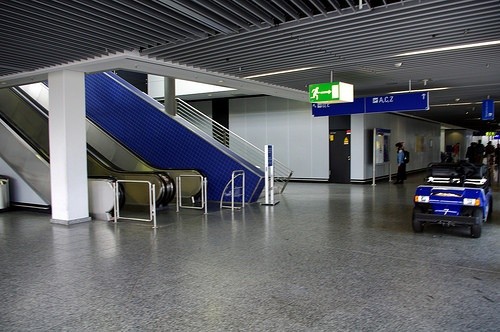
left=393, top=182, right=400, bottom=184
left=399, top=181, right=404, bottom=184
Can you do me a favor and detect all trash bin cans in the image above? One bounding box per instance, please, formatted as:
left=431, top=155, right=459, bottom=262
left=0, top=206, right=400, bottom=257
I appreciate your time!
left=0, top=175, right=10, bottom=212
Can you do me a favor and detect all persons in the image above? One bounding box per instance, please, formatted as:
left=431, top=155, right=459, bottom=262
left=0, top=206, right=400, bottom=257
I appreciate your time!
left=465, top=139, right=500, bottom=169
left=443, top=140, right=460, bottom=162
left=393, top=142, right=407, bottom=184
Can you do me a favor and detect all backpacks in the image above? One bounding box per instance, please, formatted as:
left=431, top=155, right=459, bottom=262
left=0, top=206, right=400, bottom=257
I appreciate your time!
left=397, top=149, right=409, bottom=163
